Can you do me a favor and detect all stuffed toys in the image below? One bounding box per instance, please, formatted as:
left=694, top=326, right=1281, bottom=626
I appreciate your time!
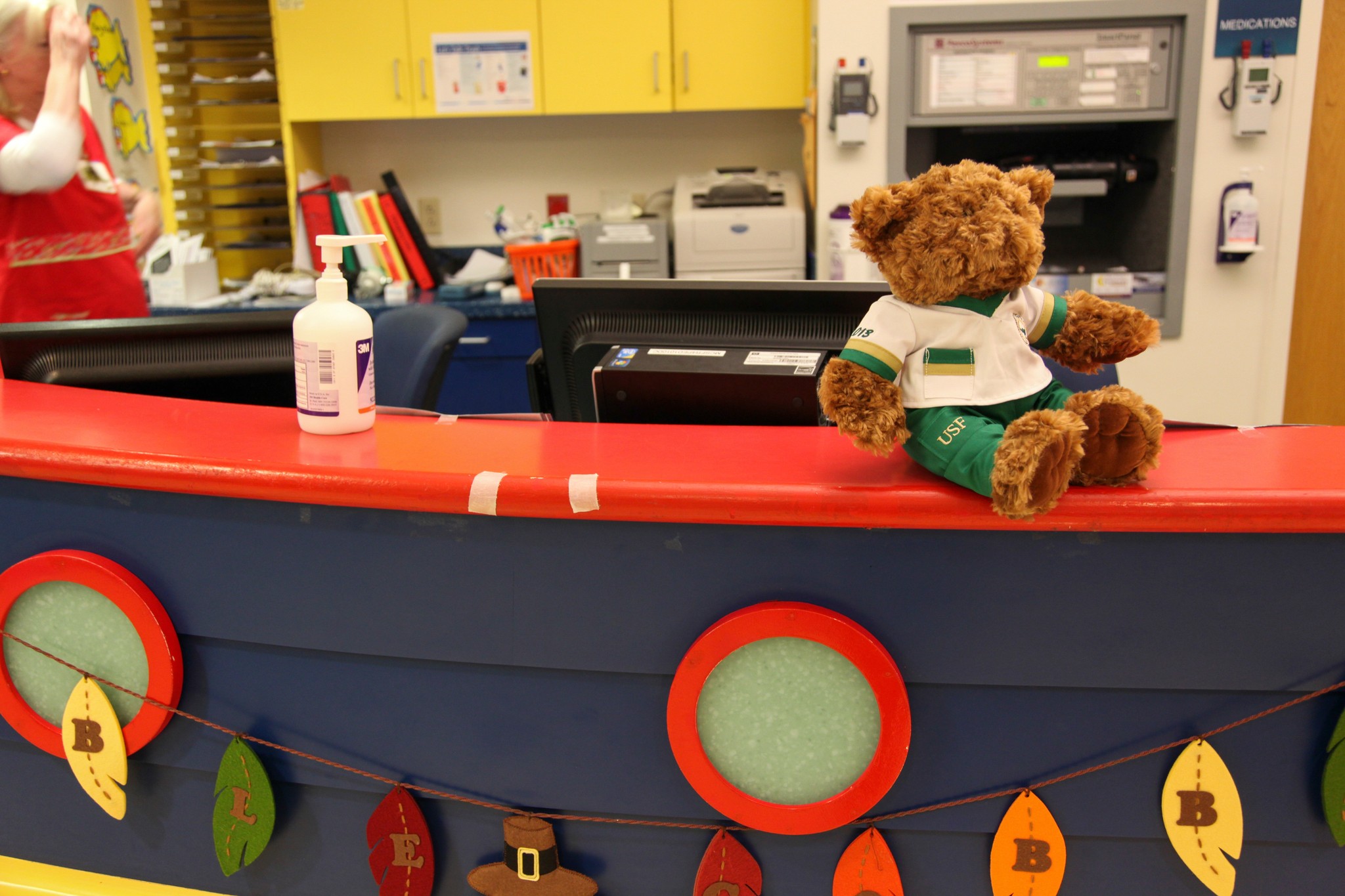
left=814, top=159, right=1164, bottom=525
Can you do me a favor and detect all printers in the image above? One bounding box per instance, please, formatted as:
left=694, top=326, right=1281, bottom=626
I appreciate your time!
left=672, top=163, right=804, bottom=281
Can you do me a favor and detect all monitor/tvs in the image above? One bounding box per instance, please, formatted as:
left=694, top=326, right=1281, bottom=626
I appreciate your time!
left=535, top=278, right=892, bottom=423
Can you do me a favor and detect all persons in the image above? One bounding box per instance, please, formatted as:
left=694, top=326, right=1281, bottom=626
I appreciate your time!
left=0, top=1, right=164, bottom=324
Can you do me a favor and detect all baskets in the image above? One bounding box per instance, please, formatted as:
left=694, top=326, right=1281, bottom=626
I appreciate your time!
left=504, top=238, right=579, bottom=300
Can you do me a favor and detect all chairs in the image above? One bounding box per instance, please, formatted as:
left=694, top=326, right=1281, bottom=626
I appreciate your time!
left=371, top=304, right=469, bottom=412
left=1038, top=354, right=1118, bottom=395
